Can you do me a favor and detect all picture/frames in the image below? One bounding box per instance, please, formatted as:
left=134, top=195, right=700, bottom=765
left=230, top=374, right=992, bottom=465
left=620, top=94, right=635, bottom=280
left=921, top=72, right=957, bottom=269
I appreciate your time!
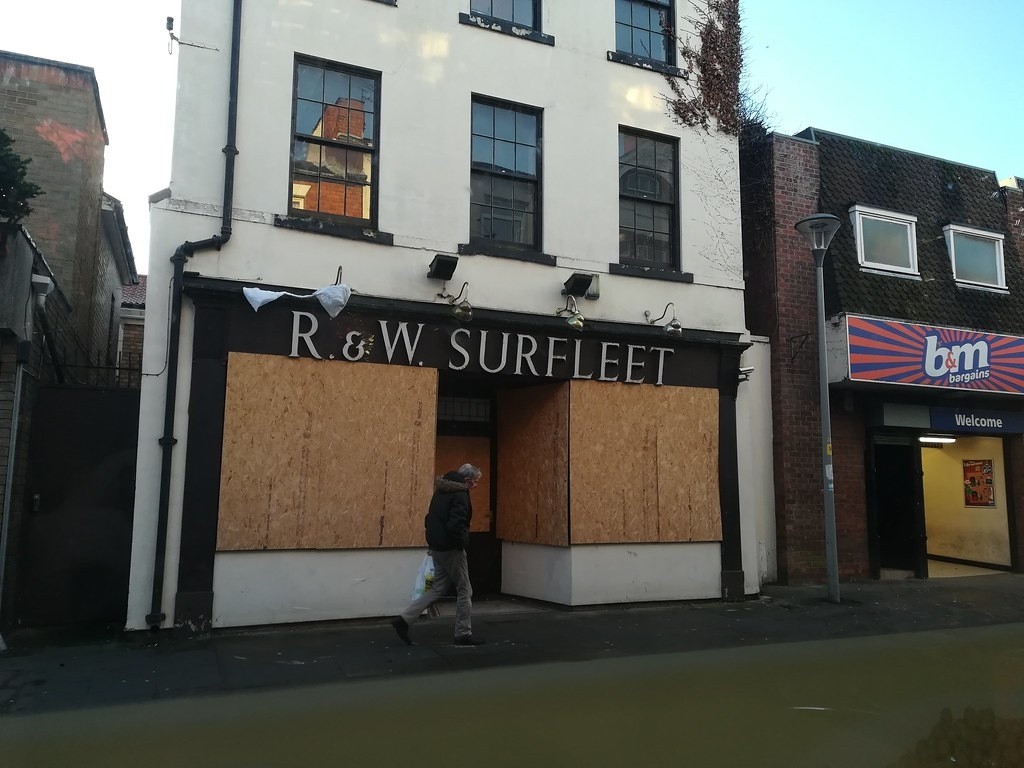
left=961, top=457, right=996, bottom=509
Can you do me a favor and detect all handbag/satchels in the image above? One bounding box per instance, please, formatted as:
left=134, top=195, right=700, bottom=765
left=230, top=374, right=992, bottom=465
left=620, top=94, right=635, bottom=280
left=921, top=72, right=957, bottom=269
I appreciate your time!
left=409, top=554, right=435, bottom=602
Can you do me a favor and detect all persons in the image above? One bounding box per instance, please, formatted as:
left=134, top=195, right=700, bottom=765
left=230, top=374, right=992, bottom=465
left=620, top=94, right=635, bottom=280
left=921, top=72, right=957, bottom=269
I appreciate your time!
left=392, top=463, right=485, bottom=646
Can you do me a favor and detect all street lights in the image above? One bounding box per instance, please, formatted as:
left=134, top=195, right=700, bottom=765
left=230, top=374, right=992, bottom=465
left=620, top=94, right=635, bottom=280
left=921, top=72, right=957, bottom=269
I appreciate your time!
left=793, top=212, right=843, bottom=604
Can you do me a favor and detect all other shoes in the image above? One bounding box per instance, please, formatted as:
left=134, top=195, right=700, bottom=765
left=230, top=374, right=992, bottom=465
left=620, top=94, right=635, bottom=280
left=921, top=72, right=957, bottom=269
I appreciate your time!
left=391, top=615, right=412, bottom=644
left=455, top=636, right=487, bottom=647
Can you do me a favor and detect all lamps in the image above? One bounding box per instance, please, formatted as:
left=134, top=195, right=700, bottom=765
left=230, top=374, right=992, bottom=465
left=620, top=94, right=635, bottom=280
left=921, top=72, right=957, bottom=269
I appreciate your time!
left=449, top=281, right=473, bottom=322
left=556, top=294, right=585, bottom=332
left=650, top=302, right=682, bottom=337
left=427, top=254, right=458, bottom=281
left=917, top=432, right=956, bottom=444
left=561, top=271, right=594, bottom=297
